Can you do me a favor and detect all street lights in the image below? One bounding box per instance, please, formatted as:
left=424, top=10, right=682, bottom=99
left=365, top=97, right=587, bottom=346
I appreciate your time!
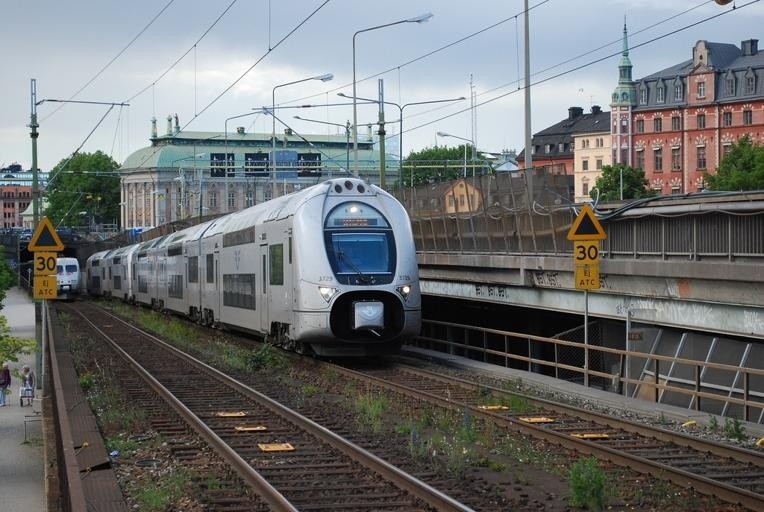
left=292, top=115, right=403, bottom=179
left=337, top=92, right=466, bottom=208
left=169, top=153, right=207, bottom=222
left=272, top=72, right=335, bottom=198
left=224, top=109, right=274, bottom=213
left=353, top=12, right=435, bottom=179
left=193, top=134, right=222, bottom=215
left=435, top=130, right=476, bottom=214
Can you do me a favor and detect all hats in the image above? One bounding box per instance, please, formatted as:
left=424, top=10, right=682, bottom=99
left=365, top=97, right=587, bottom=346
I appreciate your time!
left=0, top=223, right=118, bottom=243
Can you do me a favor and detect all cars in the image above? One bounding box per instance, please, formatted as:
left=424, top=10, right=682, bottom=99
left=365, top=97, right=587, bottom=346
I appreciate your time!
left=0, top=223, right=118, bottom=243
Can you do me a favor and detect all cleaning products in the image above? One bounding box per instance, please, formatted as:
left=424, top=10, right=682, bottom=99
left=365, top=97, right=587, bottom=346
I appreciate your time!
left=19, top=381, right=35, bottom=407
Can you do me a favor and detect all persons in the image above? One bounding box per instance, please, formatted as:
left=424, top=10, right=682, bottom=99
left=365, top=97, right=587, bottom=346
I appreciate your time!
left=21, top=365, right=35, bottom=406
left=1, top=363, right=12, bottom=407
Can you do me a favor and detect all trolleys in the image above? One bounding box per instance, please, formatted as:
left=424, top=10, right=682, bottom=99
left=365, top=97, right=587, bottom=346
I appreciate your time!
left=19, top=381, right=35, bottom=407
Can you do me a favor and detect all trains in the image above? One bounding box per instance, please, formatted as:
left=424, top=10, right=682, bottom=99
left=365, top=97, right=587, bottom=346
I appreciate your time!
left=20, top=257, right=81, bottom=300
left=85, top=180, right=423, bottom=360
